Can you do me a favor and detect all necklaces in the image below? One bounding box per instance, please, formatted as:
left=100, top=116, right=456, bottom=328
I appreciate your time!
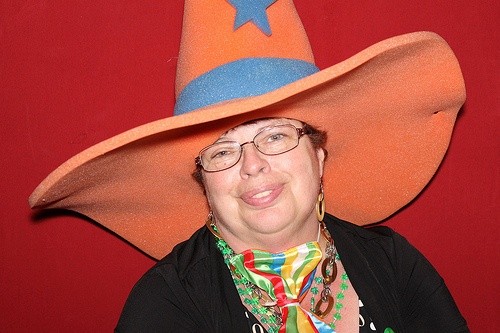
left=213, top=221, right=348, bottom=333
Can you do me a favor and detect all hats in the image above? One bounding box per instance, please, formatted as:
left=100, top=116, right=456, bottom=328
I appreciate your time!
left=28, top=0, right=466, bottom=262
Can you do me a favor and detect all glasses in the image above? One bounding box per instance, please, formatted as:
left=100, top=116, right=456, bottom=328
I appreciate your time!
left=194, top=123, right=311, bottom=174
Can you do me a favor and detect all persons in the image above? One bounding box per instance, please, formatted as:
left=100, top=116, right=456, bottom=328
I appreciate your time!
left=113, top=1, right=472, bottom=332
